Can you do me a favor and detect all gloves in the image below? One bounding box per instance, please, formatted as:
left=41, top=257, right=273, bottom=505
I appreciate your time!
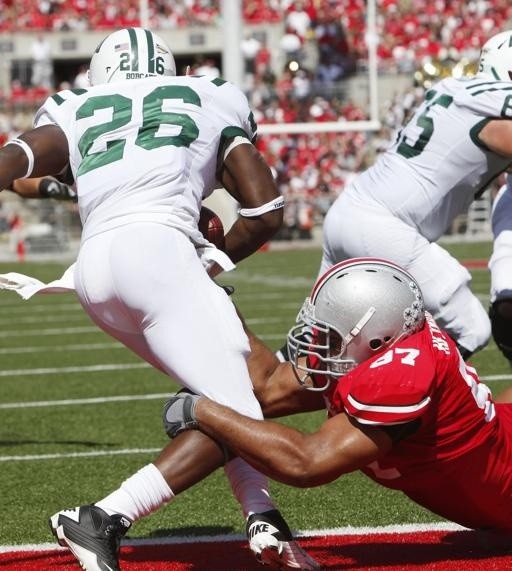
left=160, top=387, right=204, bottom=438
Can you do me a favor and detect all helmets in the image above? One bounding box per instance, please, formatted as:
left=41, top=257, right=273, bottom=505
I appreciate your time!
left=477, top=31, right=511, bottom=85
left=86, top=29, right=177, bottom=85
left=286, top=256, right=424, bottom=391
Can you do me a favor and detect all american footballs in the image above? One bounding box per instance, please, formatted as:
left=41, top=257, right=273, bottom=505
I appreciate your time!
left=196, top=205, right=225, bottom=248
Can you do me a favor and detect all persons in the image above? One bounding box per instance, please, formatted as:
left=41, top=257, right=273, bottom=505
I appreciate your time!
left=489, top=173, right=510, bottom=366
left=275, top=28, right=512, bottom=375
left=1, top=0, right=512, bottom=240
left=1, top=30, right=320, bottom=571
left=162, top=258, right=509, bottom=538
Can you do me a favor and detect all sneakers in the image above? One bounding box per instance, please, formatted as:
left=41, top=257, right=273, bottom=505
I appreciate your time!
left=49, top=501, right=131, bottom=571
left=246, top=510, right=318, bottom=571
